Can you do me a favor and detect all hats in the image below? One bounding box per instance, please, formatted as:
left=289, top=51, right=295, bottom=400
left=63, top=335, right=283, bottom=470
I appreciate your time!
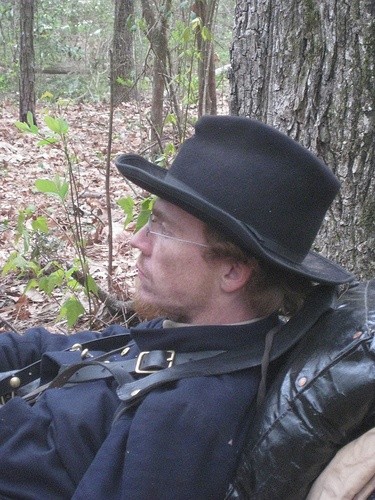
left=115, top=115, right=355, bottom=285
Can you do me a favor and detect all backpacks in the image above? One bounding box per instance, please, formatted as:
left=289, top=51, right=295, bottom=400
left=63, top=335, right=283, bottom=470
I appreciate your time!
left=0, top=276, right=375, bottom=500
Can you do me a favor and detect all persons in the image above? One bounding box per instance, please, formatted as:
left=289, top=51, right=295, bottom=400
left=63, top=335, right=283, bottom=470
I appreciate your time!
left=0, top=115, right=374, bottom=500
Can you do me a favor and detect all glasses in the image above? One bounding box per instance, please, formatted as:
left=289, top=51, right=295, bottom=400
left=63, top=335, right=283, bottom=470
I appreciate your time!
left=146, top=212, right=210, bottom=247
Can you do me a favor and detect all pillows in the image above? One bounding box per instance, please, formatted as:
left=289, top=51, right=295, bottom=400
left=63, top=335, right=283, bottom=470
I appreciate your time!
left=226, top=279, right=375, bottom=500
